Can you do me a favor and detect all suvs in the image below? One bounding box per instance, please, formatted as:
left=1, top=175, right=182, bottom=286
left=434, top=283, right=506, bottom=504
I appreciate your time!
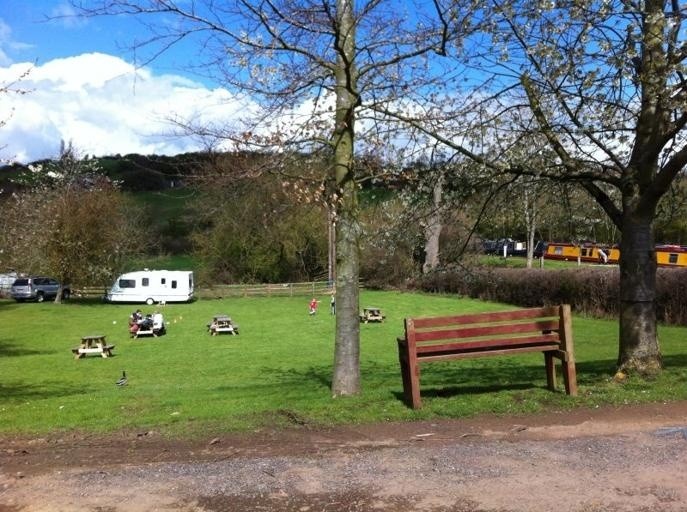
left=11, top=276, right=69, bottom=303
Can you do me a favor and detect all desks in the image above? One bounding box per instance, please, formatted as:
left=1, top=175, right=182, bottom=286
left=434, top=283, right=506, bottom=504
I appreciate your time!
left=134, top=322, right=157, bottom=339
left=72, top=334, right=114, bottom=359
left=212, top=315, right=238, bottom=336
left=359, top=308, right=386, bottom=324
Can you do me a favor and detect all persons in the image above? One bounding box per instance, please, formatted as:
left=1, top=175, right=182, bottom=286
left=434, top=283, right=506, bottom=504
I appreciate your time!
left=151, top=310, right=163, bottom=331
left=127, top=309, right=142, bottom=339
left=138, top=314, right=152, bottom=332
left=307, top=298, right=321, bottom=315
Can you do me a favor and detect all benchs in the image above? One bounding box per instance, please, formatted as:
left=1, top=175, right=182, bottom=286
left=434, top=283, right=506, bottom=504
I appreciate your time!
left=397, top=303, right=577, bottom=410
left=359, top=313, right=385, bottom=322
left=72, top=345, right=115, bottom=359
left=210, top=322, right=238, bottom=336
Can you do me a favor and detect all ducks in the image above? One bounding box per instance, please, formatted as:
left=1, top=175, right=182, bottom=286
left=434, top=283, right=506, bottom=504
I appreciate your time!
left=115, top=371, right=128, bottom=386
